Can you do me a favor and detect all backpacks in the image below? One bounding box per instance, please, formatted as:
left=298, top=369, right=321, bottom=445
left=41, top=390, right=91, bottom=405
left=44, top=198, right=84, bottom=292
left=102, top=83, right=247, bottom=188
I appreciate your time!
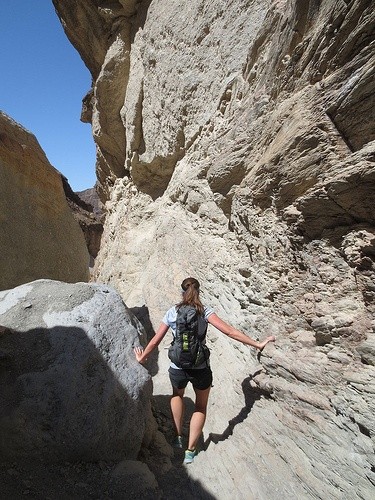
left=168, top=307, right=211, bottom=372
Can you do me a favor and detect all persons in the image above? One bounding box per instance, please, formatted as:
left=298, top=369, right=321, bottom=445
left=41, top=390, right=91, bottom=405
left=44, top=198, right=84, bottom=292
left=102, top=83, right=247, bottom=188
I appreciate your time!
left=131, top=276, right=277, bottom=468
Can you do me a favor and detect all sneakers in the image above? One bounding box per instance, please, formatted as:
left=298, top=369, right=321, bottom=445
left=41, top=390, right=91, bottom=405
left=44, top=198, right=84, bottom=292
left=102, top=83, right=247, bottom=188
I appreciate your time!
left=172, top=435, right=183, bottom=449
left=184, top=449, right=198, bottom=463
